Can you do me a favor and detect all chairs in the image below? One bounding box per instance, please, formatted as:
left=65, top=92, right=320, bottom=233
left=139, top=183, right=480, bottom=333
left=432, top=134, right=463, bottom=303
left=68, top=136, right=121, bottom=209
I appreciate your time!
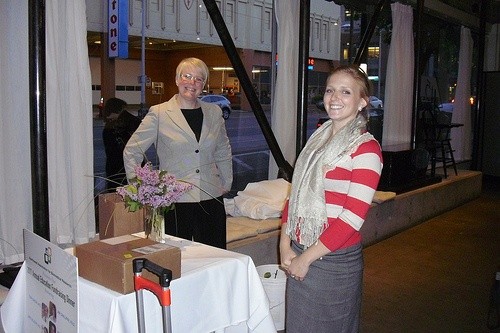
left=423, top=110, right=458, bottom=178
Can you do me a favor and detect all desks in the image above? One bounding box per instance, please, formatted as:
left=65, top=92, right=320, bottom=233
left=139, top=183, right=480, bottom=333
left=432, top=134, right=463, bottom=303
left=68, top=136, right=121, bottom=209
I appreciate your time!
left=0, top=229, right=278, bottom=333
left=429, top=121, right=464, bottom=129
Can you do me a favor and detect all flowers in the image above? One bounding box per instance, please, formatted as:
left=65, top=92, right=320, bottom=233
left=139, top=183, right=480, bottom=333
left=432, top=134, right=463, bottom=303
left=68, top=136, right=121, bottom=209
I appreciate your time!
left=67, top=134, right=235, bottom=241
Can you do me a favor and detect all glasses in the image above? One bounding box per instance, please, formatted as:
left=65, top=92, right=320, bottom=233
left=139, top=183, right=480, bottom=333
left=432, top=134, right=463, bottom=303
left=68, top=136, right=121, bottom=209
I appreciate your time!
left=181, top=73, right=206, bottom=84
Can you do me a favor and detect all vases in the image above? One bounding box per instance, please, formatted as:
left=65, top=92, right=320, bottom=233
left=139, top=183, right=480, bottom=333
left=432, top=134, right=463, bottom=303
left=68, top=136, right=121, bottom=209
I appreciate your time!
left=149, top=213, right=165, bottom=244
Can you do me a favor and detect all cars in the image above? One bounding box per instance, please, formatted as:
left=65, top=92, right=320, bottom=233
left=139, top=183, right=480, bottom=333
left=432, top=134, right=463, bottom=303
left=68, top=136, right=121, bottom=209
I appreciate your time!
left=435, top=102, right=454, bottom=117
left=198, top=94, right=232, bottom=120
left=315, top=99, right=326, bottom=112
left=368, top=95, right=384, bottom=112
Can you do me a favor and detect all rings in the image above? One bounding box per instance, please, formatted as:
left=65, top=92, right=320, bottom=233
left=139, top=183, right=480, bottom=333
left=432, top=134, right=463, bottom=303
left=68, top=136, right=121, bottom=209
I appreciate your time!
left=291, top=275, right=294, bottom=278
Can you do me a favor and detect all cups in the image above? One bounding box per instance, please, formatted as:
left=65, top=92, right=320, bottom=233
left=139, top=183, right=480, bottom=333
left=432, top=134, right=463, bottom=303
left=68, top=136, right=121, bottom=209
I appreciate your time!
left=87, top=231, right=99, bottom=242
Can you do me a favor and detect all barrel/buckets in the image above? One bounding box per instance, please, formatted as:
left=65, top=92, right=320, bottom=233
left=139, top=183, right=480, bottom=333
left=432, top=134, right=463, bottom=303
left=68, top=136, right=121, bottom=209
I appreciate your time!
left=256, top=264, right=288, bottom=332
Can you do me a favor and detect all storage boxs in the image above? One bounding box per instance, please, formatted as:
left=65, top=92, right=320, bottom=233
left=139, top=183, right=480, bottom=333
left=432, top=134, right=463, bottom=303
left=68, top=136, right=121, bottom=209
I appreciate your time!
left=96, top=191, right=147, bottom=239
left=75, top=233, right=181, bottom=296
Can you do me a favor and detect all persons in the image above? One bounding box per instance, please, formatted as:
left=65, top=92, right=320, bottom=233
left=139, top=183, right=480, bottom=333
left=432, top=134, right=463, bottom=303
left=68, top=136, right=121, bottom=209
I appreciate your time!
left=123, top=58, right=233, bottom=250
left=103, top=98, right=142, bottom=192
left=280, top=64, right=384, bottom=333
